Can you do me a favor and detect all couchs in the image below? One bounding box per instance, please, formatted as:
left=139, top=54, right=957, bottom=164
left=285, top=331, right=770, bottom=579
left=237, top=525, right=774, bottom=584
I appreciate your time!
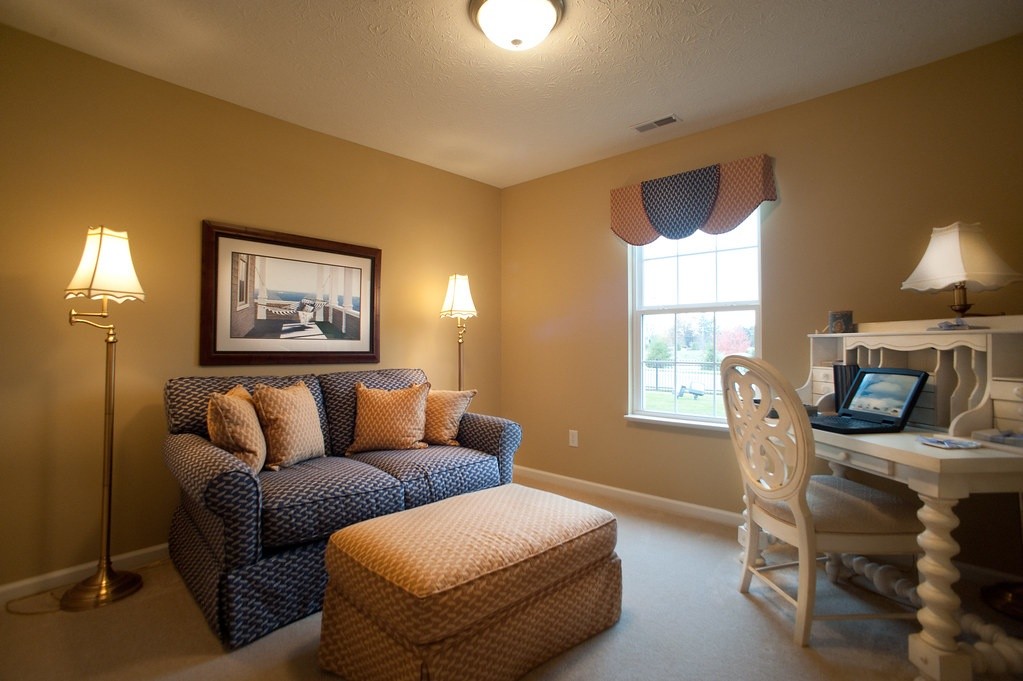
left=159, top=368, right=524, bottom=653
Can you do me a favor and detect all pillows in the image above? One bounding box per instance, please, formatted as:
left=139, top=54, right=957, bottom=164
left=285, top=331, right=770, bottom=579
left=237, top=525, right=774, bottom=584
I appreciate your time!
left=345, top=379, right=432, bottom=453
left=423, top=389, right=477, bottom=452
left=254, top=380, right=326, bottom=469
left=203, top=384, right=267, bottom=477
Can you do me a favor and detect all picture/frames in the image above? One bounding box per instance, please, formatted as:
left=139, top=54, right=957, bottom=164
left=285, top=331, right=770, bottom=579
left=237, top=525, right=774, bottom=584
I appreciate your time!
left=200, top=219, right=393, bottom=366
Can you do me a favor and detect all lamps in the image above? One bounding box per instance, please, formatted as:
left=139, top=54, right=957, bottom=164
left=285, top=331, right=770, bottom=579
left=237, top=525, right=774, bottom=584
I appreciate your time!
left=60, top=224, right=146, bottom=612
left=439, top=273, right=479, bottom=392
left=468, top=0, right=566, bottom=51
left=899, top=220, right=1023, bottom=330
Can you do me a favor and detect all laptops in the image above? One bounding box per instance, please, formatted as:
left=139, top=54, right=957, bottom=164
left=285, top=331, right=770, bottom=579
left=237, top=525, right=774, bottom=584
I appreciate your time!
left=808, top=367, right=930, bottom=434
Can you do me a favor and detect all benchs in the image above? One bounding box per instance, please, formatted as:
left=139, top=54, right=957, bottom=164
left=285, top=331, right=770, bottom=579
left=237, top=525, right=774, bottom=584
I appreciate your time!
left=319, top=482, right=621, bottom=681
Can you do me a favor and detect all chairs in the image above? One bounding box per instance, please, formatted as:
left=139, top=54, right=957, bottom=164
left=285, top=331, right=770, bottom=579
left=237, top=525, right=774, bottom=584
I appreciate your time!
left=719, top=353, right=927, bottom=649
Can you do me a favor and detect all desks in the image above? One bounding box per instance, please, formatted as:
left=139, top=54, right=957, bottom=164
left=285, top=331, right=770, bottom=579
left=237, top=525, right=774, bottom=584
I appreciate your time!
left=734, top=314, right=1023, bottom=681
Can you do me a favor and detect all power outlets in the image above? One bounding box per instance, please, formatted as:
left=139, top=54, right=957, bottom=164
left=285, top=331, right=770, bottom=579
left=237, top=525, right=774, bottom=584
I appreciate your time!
left=568, top=429, right=579, bottom=448
